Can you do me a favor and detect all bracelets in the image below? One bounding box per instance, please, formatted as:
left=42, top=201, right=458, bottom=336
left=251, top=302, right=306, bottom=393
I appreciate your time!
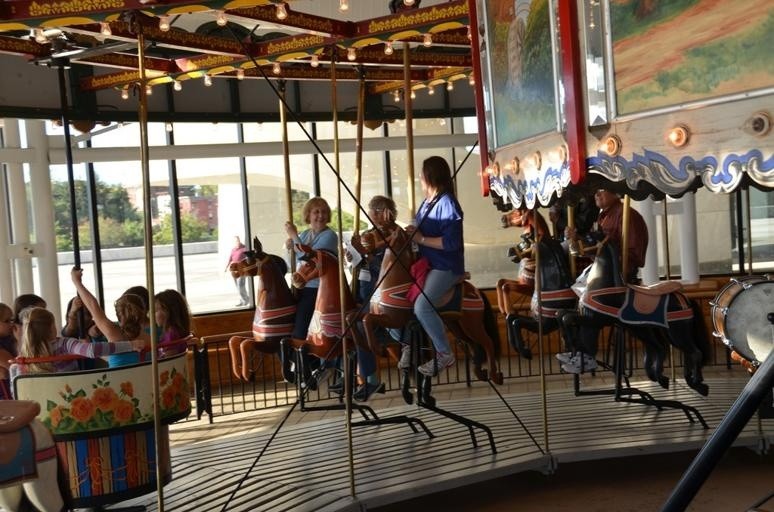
left=420, top=235, right=425, bottom=245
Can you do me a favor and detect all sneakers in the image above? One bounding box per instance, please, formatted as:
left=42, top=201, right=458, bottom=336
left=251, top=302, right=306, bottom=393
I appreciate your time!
left=416, top=350, right=457, bottom=377
left=397, top=342, right=413, bottom=369
left=352, top=380, right=384, bottom=399
left=554, top=349, right=599, bottom=376
left=326, top=375, right=345, bottom=392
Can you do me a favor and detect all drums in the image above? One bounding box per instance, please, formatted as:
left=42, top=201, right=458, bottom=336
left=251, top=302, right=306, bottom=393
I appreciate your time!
left=708, top=274, right=774, bottom=363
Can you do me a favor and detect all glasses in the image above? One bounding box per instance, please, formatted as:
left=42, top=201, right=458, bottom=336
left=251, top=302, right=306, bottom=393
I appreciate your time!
left=0, top=317, right=16, bottom=324
left=596, top=188, right=606, bottom=192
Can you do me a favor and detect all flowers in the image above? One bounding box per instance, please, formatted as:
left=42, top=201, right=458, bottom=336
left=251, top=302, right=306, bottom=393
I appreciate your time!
left=140, top=364, right=191, bottom=422
left=39, top=373, right=143, bottom=438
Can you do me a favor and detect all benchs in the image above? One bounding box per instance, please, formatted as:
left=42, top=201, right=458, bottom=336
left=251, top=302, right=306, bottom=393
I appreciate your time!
left=679, top=275, right=756, bottom=347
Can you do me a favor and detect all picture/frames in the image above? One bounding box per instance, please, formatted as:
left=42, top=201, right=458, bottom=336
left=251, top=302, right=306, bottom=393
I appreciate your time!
left=476, top=0, right=566, bottom=153
left=600, top=1, right=774, bottom=124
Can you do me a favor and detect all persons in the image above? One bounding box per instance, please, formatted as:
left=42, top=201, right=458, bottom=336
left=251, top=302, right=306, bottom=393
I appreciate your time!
left=325, top=194, right=398, bottom=399
left=286, top=195, right=339, bottom=375
left=0, top=266, right=191, bottom=401
left=556, top=188, right=649, bottom=374
left=382, top=153, right=467, bottom=376
left=223, top=234, right=251, bottom=308
left=228, top=205, right=710, bottom=399
